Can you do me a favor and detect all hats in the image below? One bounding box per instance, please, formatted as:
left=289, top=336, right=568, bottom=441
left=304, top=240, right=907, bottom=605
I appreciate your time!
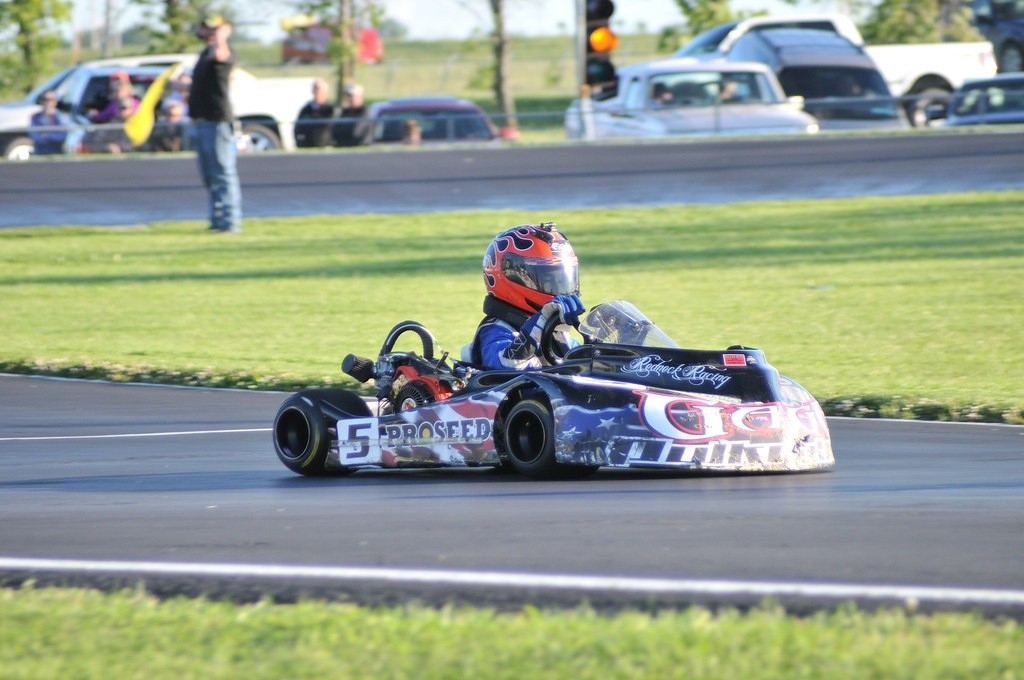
left=203, top=15, right=223, bottom=29
left=111, top=70, right=132, bottom=83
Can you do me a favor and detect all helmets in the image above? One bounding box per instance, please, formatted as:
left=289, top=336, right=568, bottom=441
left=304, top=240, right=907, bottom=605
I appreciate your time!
left=482, top=222, right=580, bottom=336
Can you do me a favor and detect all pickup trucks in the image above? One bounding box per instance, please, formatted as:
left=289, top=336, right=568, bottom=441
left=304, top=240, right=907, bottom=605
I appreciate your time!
left=671, top=10, right=1000, bottom=128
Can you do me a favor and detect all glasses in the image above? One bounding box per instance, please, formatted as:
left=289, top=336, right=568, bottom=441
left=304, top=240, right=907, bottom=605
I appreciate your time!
left=178, top=83, right=190, bottom=92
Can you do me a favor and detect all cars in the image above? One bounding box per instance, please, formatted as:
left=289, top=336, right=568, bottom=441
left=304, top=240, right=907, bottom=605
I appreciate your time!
left=334, top=97, right=521, bottom=147
left=924, top=69, right=1024, bottom=126
left=562, top=57, right=819, bottom=139
left=1, top=52, right=326, bottom=164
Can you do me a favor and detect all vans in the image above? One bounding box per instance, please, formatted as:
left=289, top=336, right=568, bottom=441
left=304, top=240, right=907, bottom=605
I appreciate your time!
left=728, top=26, right=913, bottom=131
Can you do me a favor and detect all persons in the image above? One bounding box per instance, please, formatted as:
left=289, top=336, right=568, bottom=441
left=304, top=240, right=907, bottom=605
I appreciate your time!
left=470, top=223, right=651, bottom=375
left=30, top=68, right=372, bottom=149
left=650, top=77, right=746, bottom=106
left=187, top=13, right=243, bottom=232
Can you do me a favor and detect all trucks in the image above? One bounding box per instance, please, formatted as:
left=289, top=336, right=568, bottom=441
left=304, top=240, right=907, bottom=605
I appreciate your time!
left=969, top=0, right=1024, bottom=76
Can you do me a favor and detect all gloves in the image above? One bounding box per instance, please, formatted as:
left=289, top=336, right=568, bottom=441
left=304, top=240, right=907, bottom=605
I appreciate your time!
left=519, top=294, right=585, bottom=350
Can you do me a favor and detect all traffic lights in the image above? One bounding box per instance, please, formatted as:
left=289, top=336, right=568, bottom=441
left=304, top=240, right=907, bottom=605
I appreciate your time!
left=574, top=0, right=620, bottom=102
